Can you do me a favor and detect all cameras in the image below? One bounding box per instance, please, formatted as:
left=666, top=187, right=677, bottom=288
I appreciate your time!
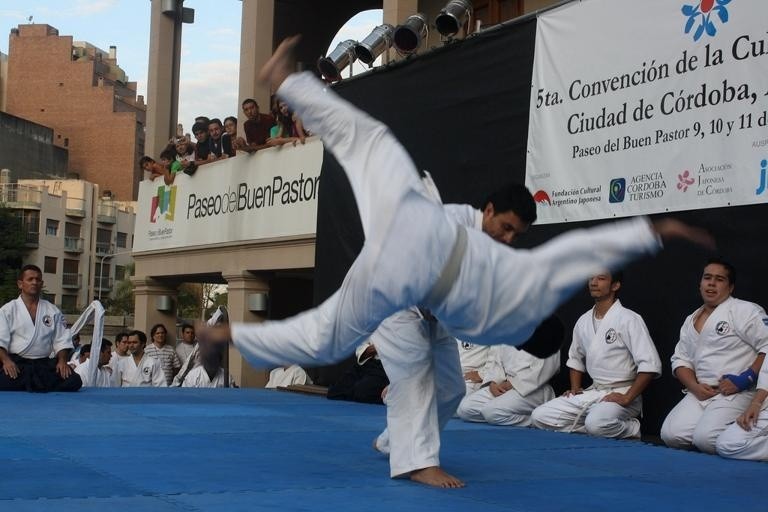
left=174, top=138, right=183, bottom=144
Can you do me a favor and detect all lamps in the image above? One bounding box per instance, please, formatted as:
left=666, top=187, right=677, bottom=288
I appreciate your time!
left=314, top=1, right=475, bottom=85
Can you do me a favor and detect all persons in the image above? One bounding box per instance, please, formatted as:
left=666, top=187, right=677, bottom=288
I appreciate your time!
left=358, top=184, right=536, bottom=487
left=265, top=362, right=313, bottom=388
left=66, top=322, right=225, bottom=388
left=139, top=95, right=306, bottom=185
left=660, top=259, right=767, bottom=460
left=0, top=264, right=83, bottom=393
left=195, top=36, right=719, bottom=370
left=451, top=316, right=563, bottom=431
left=530, top=262, right=662, bottom=441
